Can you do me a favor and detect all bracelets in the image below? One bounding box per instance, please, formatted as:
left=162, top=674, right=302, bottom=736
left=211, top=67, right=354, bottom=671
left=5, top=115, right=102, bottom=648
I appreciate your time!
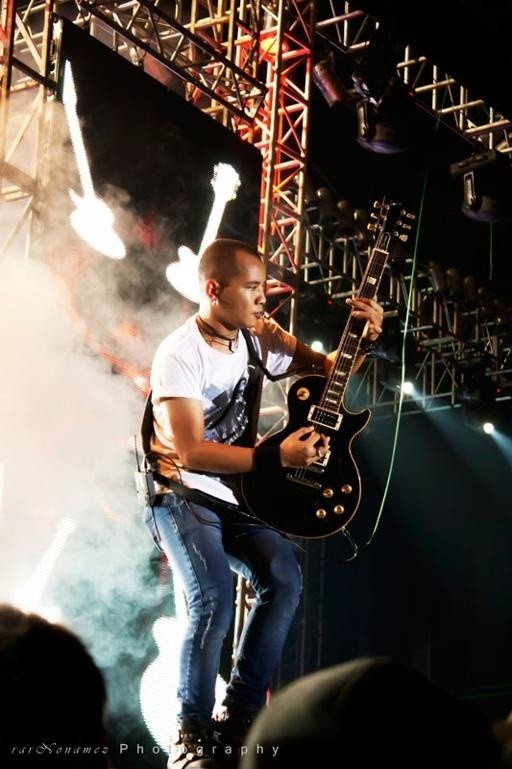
left=254, top=445, right=284, bottom=471
left=360, top=336, right=378, bottom=356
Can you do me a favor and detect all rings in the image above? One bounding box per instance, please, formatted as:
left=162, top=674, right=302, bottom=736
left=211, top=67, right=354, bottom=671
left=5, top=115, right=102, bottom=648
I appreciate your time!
left=315, top=448, right=327, bottom=458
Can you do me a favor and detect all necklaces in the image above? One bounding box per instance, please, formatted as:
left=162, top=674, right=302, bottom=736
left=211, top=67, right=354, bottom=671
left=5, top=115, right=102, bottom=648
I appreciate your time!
left=196, top=314, right=239, bottom=354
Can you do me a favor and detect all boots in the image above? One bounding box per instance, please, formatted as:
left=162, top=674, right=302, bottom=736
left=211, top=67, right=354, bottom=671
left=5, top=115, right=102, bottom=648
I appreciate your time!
left=167, top=700, right=252, bottom=769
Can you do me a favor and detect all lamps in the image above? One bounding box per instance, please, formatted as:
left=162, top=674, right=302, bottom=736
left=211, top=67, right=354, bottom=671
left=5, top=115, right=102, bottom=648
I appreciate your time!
left=451, top=149, right=503, bottom=223
left=313, top=21, right=412, bottom=153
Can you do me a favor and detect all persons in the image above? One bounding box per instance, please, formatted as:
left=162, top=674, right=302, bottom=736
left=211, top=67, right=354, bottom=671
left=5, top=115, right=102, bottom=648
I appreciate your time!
left=0, top=602, right=123, bottom=768
left=241, top=648, right=504, bottom=768
left=132, top=237, right=388, bottom=768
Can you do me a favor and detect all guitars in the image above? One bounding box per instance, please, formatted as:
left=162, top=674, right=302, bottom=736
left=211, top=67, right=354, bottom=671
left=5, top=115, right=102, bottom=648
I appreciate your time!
left=243, top=202, right=416, bottom=539
left=63, top=60, right=127, bottom=261
left=165, top=162, right=240, bottom=304
left=139, top=567, right=228, bottom=760
